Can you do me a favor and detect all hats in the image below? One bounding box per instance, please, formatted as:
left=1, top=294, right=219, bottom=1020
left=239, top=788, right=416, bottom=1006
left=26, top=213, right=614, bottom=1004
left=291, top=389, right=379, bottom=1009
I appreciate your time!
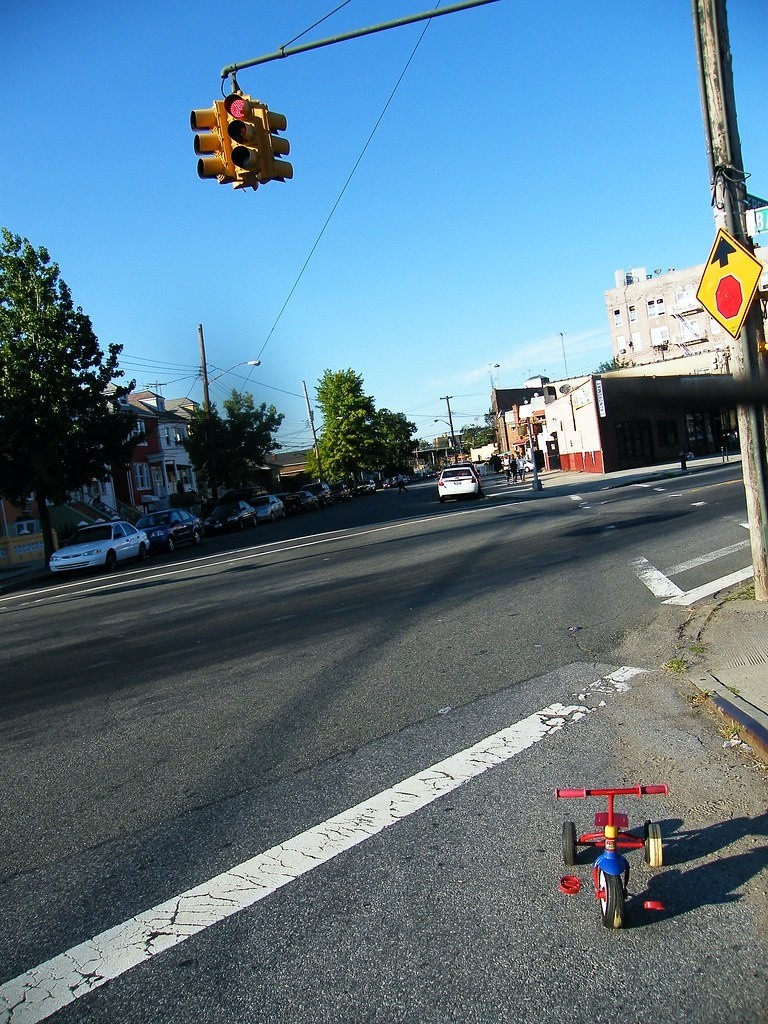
left=517, top=455, right=521, bottom=457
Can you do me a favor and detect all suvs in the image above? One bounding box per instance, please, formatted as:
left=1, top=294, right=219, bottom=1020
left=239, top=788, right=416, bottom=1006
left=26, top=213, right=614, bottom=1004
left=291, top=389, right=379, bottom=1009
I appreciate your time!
left=436, top=463, right=484, bottom=504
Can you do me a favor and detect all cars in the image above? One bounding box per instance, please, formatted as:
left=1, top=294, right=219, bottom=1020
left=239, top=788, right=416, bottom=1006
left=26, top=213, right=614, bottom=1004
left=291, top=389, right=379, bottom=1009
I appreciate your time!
left=250, top=495, right=286, bottom=523
left=49, top=520, right=151, bottom=574
left=382, top=473, right=437, bottom=489
left=276, top=480, right=376, bottom=512
left=512, top=459, right=534, bottom=473
left=134, top=509, right=203, bottom=554
left=203, top=500, right=256, bottom=536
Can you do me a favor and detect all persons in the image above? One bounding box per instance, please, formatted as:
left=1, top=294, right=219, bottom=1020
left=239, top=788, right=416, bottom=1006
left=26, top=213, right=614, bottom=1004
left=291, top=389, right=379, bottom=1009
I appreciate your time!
left=505, top=457, right=526, bottom=484
left=177, top=480, right=185, bottom=493
left=93, top=495, right=105, bottom=512
left=395, top=472, right=409, bottom=495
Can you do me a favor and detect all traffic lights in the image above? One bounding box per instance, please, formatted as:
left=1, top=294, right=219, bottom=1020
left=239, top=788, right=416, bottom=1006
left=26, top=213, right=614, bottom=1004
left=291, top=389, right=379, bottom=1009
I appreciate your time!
left=222, top=92, right=266, bottom=173
left=262, top=110, right=293, bottom=181
left=189, top=100, right=229, bottom=183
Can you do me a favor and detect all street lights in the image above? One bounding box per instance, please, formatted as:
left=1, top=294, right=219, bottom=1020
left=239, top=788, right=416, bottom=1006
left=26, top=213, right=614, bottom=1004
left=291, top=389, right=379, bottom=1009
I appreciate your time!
left=203, top=360, right=262, bottom=421
left=312, top=416, right=345, bottom=483
left=435, top=419, right=457, bottom=463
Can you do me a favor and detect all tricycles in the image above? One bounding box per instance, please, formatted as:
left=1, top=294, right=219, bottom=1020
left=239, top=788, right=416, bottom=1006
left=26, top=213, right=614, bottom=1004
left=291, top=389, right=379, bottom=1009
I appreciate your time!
left=550, top=785, right=666, bottom=927
